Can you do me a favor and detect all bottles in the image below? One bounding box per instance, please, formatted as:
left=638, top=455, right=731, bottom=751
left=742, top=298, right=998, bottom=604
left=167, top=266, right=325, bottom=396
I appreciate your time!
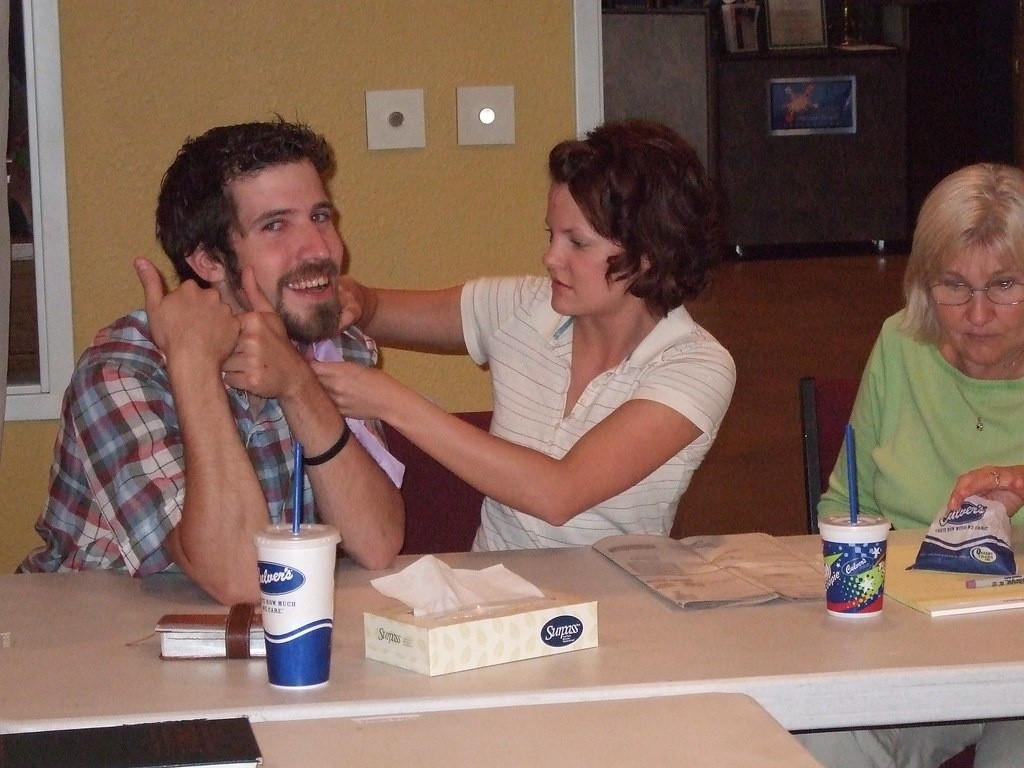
left=839, top=2, right=852, bottom=43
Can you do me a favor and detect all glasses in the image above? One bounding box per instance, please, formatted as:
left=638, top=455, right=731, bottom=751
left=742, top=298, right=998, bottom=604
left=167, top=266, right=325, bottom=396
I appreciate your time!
left=927, top=278, right=1024, bottom=306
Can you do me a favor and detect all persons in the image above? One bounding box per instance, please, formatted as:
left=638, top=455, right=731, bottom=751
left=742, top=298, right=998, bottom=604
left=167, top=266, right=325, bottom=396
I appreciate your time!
left=817, top=162, right=1024, bottom=768
left=15, top=113, right=403, bottom=604
left=310, top=119, right=737, bottom=554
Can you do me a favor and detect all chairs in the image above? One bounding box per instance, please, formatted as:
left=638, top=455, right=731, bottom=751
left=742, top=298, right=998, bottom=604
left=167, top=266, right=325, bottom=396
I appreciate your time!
left=800, top=375, right=975, bottom=768
left=374, top=410, right=496, bottom=556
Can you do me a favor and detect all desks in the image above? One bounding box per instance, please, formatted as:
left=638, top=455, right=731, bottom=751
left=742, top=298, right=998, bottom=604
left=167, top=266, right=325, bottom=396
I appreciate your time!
left=0, top=524, right=1024, bottom=768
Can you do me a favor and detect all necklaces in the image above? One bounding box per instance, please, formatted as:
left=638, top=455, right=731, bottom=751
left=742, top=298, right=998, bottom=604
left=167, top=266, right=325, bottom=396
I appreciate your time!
left=954, top=360, right=1016, bottom=430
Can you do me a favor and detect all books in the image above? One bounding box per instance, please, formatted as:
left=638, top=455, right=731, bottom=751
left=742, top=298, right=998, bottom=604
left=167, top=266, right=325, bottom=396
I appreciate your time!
left=817, top=543, right=1024, bottom=617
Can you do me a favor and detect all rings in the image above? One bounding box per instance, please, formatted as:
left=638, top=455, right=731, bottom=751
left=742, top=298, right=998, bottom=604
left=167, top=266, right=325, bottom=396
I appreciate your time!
left=993, top=472, right=1000, bottom=490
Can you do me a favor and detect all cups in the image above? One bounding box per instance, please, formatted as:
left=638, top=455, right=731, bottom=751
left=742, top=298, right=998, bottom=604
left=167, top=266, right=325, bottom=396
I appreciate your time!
left=252, top=523, right=343, bottom=689
left=817, top=514, right=891, bottom=618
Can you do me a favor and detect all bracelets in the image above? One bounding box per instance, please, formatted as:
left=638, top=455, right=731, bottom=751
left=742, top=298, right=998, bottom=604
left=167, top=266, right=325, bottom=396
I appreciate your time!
left=302, top=420, right=350, bottom=466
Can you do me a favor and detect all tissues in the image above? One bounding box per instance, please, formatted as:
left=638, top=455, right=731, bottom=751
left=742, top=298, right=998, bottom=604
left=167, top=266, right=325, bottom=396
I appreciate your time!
left=363, top=554, right=598, bottom=677
